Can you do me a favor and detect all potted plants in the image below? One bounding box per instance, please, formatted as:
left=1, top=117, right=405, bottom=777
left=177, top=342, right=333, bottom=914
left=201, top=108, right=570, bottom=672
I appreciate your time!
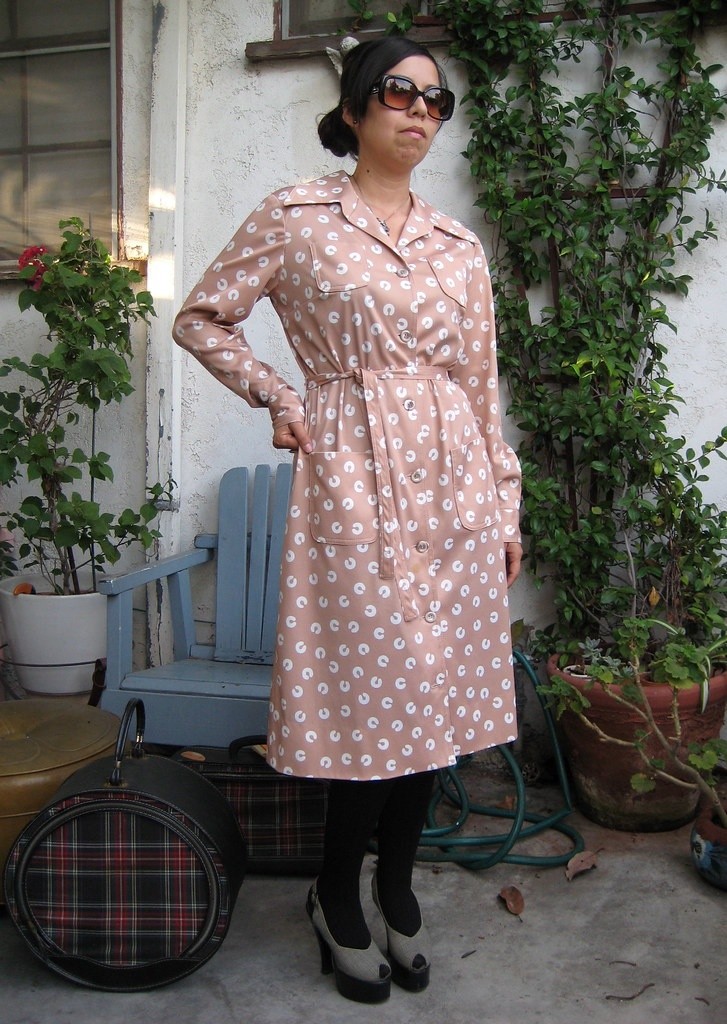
left=547, top=483, right=727, bottom=834
left=0, top=216, right=177, bottom=695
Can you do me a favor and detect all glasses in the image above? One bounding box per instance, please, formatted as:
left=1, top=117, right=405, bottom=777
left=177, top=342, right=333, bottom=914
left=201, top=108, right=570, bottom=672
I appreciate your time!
left=369, top=74, right=455, bottom=121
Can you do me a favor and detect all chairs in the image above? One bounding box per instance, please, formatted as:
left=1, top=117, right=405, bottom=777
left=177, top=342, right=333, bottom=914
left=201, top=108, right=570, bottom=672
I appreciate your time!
left=100, top=463, right=294, bottom=748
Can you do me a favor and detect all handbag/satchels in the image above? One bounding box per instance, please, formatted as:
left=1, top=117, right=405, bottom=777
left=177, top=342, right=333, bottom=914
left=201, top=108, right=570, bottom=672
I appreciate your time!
left=4, top=697, right=248, bottom=993
left=176, top=734, right=329, bottom=879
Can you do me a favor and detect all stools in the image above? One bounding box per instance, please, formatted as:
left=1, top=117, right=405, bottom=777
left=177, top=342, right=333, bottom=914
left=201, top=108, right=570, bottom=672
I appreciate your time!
left=0, top=699, right=134, bottom=904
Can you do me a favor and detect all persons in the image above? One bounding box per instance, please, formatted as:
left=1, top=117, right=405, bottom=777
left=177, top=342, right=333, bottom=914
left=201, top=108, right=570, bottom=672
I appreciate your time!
left=171, top=38, right=524, bottom=1004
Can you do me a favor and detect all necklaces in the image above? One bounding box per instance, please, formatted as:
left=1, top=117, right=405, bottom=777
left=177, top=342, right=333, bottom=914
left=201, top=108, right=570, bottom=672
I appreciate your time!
left=351, top=173, right=409, bottom=234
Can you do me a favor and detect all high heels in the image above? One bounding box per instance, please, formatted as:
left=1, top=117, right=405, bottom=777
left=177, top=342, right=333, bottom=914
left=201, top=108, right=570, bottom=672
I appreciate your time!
left=372, top=871, right=431, bottom=993
left=306, top=875, right=391, bottom=1005
